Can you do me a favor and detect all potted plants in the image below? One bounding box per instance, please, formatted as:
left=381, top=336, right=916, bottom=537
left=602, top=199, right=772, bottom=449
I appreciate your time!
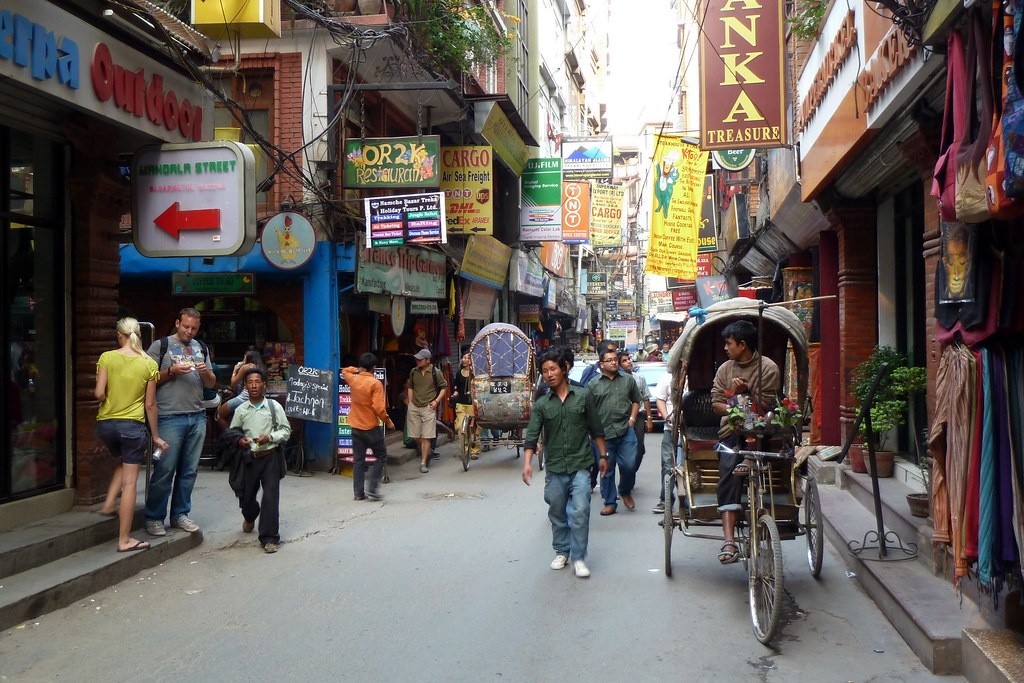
left=847, top=344, right=911, bottom=472
left=854, top=400, right=908, bottom=477
left=890, top=365, right=930, bottom=519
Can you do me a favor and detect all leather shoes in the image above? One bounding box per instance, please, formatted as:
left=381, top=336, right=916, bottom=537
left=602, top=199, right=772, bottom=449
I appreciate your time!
left=600, top=504, right=616, bottom=515
left=623, top=495, right=635, bottom=509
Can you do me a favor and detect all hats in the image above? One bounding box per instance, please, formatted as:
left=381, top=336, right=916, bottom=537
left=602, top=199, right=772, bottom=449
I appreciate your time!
left=413, top=349, right=431, bottom=360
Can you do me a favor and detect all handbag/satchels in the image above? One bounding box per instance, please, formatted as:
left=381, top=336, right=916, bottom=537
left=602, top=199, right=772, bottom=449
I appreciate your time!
left=278, top=443, right=287, bottom=479
left=930, top=95, right=1024, bottom=224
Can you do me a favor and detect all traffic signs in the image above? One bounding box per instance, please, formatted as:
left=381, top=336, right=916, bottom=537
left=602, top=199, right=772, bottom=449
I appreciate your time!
left=131, top=141, right=256, bottom=258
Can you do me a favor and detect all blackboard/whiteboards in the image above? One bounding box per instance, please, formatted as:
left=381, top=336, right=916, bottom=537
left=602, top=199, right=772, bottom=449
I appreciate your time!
left=285, top=363, right=333, bottom=423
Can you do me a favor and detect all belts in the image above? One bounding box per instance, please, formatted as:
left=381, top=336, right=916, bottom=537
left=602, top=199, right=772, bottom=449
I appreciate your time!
left=252, top=447, right=279, bottom=458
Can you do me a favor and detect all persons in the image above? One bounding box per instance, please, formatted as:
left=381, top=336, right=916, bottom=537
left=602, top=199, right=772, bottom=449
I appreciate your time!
left=341, top=353, right=396, bottom=501
left=710, top=321, right=781, bottom=564
left=633, top=341, right=670, bottom=362
left=652, top=374, right=688, bottom=513
left=229, top=369, right=292, bottom=553
left=218, top=351, right=264, bottom=431
left=204, top=344, right=220, bottom=408
left=523, top=352, right=609, bottom=576
left=141, top=307, right=215, bottom=535
left=581, top=339, right=620, bottom=500
left=398, top=349, right=500, bottom=473
left=617, top=352, right=652, bottom=490
left=94, top=317, right=170, bottom=553
left=535, top=347, right=584, bottom=402
left=588, top=348, right=642, bottom=515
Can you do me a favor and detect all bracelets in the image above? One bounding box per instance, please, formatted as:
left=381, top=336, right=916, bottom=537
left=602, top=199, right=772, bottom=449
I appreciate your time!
left=268, top=435, right=273, bottom=443
left=435, top=400, right=438, bottom=404
left=647, top=416, right=652, bottom=418
left=168, top=365, right=175, bottom=376
left=630, top=417, right=636, bottom=423
left=600, top=455, right=607, bottom=460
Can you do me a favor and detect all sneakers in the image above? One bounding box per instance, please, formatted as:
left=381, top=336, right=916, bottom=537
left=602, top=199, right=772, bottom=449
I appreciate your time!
left=171, top=515, right=199, bottom=532
left=243, top=519, right=255, bottom=532
left=146, top=520, right=166, bottom=536
left=652, top=502, right=665, bottom=513
left=550, top=554, right=567, bottom=570
left=575, top=560, right=590, bottom=577
left=264, top=542, right=278, bottom=553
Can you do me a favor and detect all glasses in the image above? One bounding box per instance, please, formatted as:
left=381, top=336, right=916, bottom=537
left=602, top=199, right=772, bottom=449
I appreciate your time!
left=603, top=358, right=619, bottom=363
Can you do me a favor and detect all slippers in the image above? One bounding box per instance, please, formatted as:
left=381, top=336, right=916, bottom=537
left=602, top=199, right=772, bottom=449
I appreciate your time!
left=101, top=508, right=120, bottom=516
left=117, top=540, right=151, bottom=552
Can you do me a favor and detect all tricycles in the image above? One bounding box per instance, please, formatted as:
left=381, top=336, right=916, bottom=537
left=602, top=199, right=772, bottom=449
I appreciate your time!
left=460, top=323, right=544, bottom=471
left=658, top=295, right=824, bottom=644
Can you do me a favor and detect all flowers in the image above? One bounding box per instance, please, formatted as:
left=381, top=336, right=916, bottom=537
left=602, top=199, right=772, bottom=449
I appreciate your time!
left=770, top=393, right=805, bottom=452
left=726, top=393, right=767, bottom=441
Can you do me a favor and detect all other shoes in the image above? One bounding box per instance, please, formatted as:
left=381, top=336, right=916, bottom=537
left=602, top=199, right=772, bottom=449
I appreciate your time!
left=365, top=491, right=383, bottom=501
left=354, top=491, right=368, bottom=501
left=426, top=455, right=431, bottom=467
left=420, top=464, right=429, bottom=473
left=430, top=452, right=441, bottom=459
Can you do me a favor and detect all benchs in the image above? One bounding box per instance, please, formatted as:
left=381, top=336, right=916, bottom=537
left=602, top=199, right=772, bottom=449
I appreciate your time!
left=679, top=389, right=796, bottom=487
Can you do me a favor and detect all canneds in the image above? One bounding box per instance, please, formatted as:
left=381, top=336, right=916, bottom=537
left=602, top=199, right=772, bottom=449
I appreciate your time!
left=153, top=445, right=163, bottom=460
left=282, top=359, right=287, bottom=366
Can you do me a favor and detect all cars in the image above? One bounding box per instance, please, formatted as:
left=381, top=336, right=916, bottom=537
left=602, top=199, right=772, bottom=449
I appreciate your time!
left=568, top=358, right=667, bottom=422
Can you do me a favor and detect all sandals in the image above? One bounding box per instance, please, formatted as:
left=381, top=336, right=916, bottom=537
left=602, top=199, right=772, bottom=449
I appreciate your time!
left=732, top=454, right=756, bottom=476
left=718, top=541, right=740, bottom=564
